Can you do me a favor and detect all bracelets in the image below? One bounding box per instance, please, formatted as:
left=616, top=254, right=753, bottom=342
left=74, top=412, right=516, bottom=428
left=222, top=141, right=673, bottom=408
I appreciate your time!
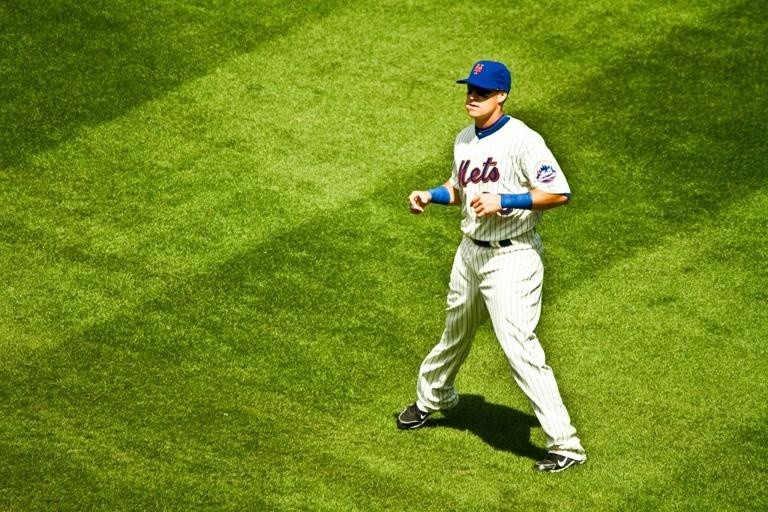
left=426, top=185, right=450, bottom=205
left=498, top=192, right=533, bottom=209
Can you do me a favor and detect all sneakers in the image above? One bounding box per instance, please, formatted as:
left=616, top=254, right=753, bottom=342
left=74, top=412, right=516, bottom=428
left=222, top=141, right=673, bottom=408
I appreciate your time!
left=533, top=451, right=587, bottom=473
left=396, top=395, right=459, bottom=429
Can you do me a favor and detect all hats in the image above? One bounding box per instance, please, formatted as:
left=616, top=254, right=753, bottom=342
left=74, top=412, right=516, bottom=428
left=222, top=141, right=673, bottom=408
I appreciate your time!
left=455, top=59, right=511, bottom=92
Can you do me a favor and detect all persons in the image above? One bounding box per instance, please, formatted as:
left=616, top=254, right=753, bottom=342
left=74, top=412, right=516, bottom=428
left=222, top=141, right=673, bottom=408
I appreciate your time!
left=398, top=60, right=589, bottom=473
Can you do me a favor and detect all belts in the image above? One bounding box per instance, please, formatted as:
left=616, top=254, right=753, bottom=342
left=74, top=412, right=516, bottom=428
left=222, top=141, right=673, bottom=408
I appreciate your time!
left=468, top=238, right=510, bottom=248
